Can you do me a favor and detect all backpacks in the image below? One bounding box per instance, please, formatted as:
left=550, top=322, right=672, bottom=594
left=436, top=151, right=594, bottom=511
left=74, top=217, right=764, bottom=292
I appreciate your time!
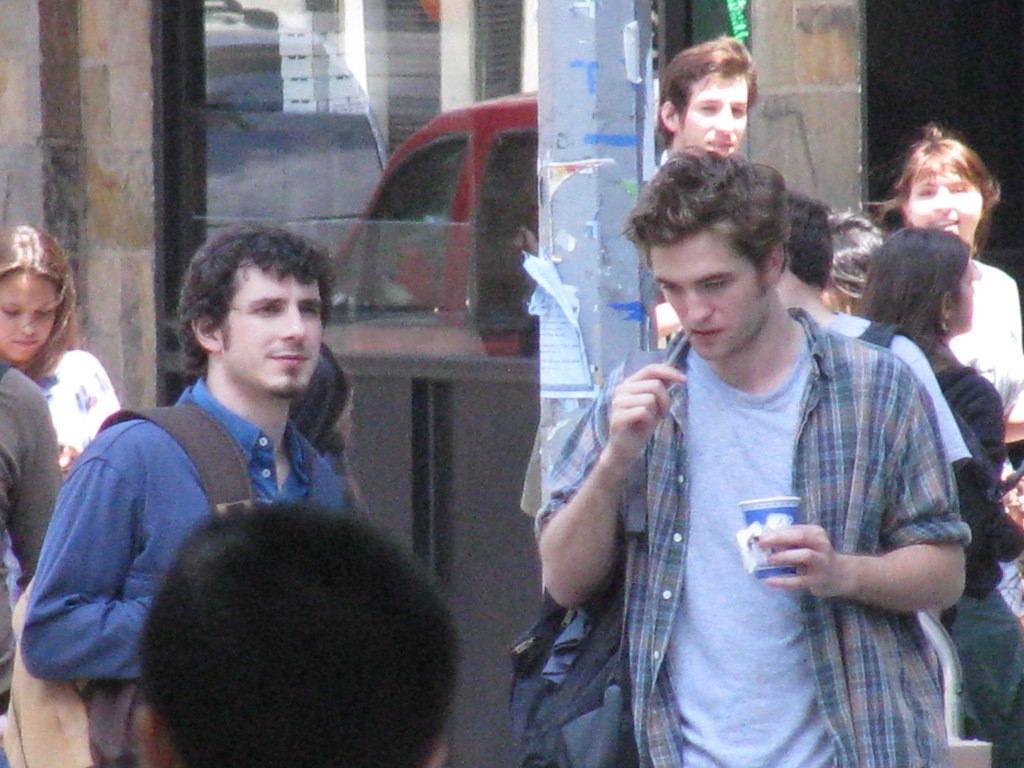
left=0, top=398, right=361, bottom=768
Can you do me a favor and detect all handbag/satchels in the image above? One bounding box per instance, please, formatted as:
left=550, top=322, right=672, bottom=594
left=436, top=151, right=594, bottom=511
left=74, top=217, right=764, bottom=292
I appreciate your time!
left=508, top=581, right=633, bottom=767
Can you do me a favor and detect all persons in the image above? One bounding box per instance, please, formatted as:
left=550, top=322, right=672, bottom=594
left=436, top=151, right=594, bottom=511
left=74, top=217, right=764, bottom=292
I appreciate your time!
left=8, top=223, right=354, bottom=768
left=0, top=359, right=62, bottom=768
left=533, top=142, right=970, bottom=767
left=524, top=32, right=1024, bottom=768
left=132, top=497, right=463, bottom=768
left=0, top=219, right=122, bottom=612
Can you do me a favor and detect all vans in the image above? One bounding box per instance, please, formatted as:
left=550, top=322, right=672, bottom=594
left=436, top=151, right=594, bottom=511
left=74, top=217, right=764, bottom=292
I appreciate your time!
left=203, top=26, right=389, bottom=311
left=344, top=99, right=539, bottom=361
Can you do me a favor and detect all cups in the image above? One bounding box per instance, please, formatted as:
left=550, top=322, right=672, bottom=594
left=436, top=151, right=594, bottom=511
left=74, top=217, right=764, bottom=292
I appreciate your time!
left=738, top=496, right=802, bottom=579
